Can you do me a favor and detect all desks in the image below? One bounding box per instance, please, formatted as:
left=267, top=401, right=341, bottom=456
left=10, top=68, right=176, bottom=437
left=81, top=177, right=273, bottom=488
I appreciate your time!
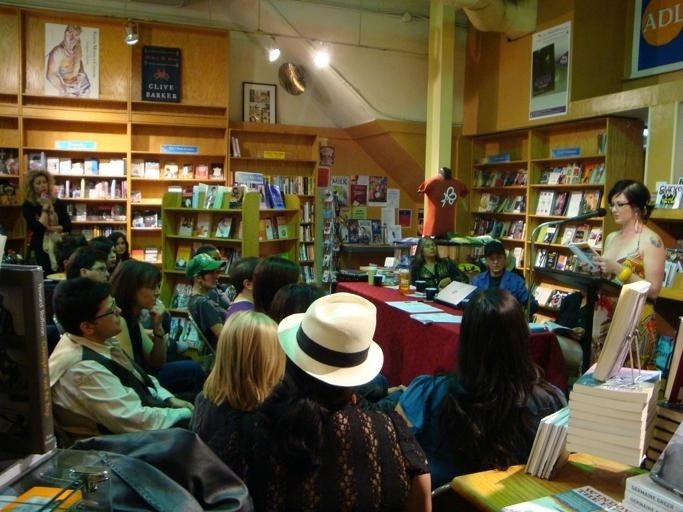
left=450, top=454, right=648, bottom=512
left=335, top=280, right=567, bottom=399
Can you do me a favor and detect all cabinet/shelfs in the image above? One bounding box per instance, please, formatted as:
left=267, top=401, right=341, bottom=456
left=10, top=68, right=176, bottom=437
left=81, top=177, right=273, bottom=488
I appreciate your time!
left=468, top=116, right=644, bottom=323
left=338, top=240, right=415, bottom=281
left=229, top=129, right=319, bottom=284
left=0, top=0, right=229, bottom=265
left=633, top=189, right=683, bottom=470
left=0, top=448, right=112, bottom=512
left=159, top=190, right=299, bottom=316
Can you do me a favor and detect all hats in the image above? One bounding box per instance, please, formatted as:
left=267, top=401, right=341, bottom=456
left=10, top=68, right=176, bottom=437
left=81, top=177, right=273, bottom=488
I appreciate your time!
left=277, top=289, right=385, bottom=389
left=483, top=240, right=506, bottom=259
left=184, top=252, right=227, bottom=281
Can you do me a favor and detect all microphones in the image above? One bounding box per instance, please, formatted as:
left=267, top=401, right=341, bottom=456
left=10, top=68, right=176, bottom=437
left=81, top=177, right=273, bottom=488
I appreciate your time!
left=559, top=207, right=608, bottom=224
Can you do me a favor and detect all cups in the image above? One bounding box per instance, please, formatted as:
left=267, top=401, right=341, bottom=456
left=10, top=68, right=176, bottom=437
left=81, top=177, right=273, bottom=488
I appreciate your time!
left=374, top=276, right=382, bottom=286
left=400, top=268, right=409, bottom=292
left=415, top=280, right=426, bottom=291
left=426, top=288, right=437, bottom=299
left=367, top=267, right=378, bottom=285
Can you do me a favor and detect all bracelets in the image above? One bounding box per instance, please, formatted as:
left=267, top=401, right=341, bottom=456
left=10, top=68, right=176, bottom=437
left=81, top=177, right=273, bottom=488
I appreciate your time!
left=617, top=268, right=631, bottom=283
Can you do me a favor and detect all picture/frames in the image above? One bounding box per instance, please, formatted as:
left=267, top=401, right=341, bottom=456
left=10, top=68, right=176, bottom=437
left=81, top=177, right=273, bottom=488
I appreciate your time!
left=242, top=82, right=278, bottom=125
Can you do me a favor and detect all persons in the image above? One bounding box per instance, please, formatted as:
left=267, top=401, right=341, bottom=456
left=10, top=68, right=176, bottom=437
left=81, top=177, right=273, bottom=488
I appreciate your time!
left=410, top=235, right=468, bottom=301
left=21, top=168, right=73, bottom=277
left=578, top=178, right=666, bottom=370
left=46, top=230, right=433, bottom=512
left=415, top=167, right=470, bottom=239
left=46, top=24, right=91, bottom=98
left=462, top=240, right=539, bottom=315
left=392, top=289, right=570, bottom=512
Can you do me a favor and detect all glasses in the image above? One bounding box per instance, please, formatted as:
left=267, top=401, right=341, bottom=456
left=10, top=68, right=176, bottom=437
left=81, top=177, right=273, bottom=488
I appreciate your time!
left=142, top=283, right=162, bottom=291
left=85, top=266, right=110, bottom=276
left=607, top=201, right=632, bottom=210
left=85, top=296, right=117, bottom=323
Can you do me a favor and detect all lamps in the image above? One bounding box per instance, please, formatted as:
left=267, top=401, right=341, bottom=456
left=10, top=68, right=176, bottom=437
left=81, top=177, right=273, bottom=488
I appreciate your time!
left=123, top=17, right=139, bottom=45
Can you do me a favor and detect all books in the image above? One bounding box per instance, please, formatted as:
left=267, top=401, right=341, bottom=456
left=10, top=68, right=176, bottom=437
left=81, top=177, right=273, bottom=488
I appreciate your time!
left=27, top=137, right=243, bottom=182
left=0, top=181, right=19, bottom=205
left=47, top=174, right=401, bottom=349
left=661, top=247, right=683, bottom=287
left=473, top=165, right=605, bottom=275
left=650, top=176, right=683, bottom=209
left=0, top=486, right=84, bottom=512
left=0, top=261, right=58, bottom=456
left=501, top=279, right=683, bottom=512
left=318, top=144, right=336, bottom=166
left=0, top=436, right=58, bottom=490
left=0, top=149, right=19, bottom=176
